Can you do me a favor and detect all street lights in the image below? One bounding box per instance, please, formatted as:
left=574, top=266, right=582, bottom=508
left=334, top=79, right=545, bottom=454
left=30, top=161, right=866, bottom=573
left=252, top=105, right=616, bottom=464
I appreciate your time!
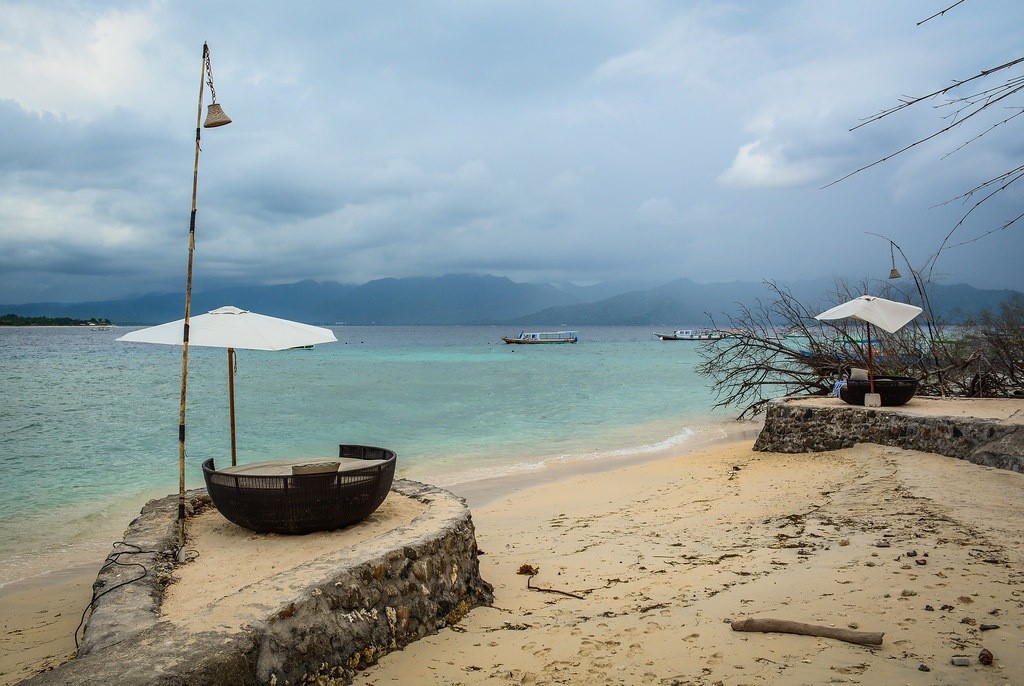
left=174, top=40, right=233, bottom=563
left=888, top=240, right=947, bottom=401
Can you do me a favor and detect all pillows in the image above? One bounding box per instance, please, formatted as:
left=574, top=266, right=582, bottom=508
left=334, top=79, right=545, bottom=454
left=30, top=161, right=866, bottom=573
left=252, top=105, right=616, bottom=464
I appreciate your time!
left=289, top=462, right=342, bottom=486
left=850, top=368, right=868, bottom=380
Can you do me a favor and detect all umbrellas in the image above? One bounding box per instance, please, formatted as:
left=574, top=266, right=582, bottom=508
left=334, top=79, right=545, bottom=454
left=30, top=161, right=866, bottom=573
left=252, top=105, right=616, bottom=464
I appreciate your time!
left=116, top=305, right=338, bottom=467
left=814, top=293, right=923, bottom=393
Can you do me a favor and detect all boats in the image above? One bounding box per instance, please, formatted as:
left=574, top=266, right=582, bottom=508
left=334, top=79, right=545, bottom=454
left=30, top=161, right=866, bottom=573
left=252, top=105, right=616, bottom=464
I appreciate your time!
left=765, top=328, right=1006, bottom=369
left=652, top=329, right=731, bottom=341
left=500, top=328, right=579, bottom=345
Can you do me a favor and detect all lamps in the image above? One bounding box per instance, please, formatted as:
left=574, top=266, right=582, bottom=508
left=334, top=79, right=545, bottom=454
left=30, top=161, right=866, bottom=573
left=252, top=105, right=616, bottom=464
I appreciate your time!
left=889, top=241, right=901, bottom=278
left=203, top=45, right=231, bottom=128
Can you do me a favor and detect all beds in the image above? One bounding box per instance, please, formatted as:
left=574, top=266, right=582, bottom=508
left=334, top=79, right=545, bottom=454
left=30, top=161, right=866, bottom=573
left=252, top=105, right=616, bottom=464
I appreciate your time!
left=201, top=444, right=398, bottom=534
left=840, top=376, right=918, bottom=406
